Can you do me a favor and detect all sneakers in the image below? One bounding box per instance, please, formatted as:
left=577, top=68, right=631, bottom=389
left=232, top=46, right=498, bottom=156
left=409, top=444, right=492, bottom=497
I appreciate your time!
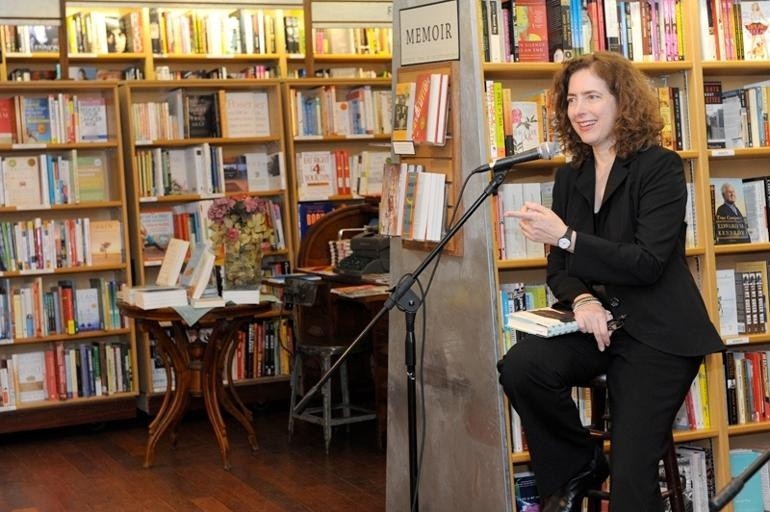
left=538, top=448, right=610, bottom=512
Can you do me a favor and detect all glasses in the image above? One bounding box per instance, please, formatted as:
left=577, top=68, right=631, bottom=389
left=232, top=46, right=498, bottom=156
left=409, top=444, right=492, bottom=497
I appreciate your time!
left=607, top=311, right=629, bottom=332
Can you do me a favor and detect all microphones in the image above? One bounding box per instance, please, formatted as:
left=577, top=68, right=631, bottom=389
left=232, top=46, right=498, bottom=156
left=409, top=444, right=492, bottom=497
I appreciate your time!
left=474, top=139, right=557, bottom=174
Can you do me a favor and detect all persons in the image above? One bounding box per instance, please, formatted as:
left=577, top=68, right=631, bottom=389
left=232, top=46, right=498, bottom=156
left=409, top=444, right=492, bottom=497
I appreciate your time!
left=496, top=50, right=726, bottom=512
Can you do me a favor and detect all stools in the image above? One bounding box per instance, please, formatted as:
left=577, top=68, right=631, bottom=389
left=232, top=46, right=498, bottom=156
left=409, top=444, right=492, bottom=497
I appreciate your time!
left=288, top=336, right=383, bottom=454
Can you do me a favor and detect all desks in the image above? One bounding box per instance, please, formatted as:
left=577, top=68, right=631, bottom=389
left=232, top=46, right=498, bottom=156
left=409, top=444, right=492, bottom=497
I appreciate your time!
left=115, top=296, right=272, bottom=471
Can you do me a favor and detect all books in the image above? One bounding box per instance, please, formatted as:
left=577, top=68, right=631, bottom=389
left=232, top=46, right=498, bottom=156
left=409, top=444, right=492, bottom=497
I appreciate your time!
left=281, top=16, right=448, bottom=295
left=507, top=308, right=612, bottom=338
left=486, top=80, right=577, bottom=358
left=506, top=387, right=610, bottom=512
left=649, top=79, right=770, bottom=512
left=0, top=9, right=288, bottom=414
left=480, top=1, right=768, bottom=59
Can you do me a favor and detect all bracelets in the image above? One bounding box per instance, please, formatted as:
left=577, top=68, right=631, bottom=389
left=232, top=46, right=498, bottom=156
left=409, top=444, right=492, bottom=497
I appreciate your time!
left=571, top=297, right=602, bottom=314
